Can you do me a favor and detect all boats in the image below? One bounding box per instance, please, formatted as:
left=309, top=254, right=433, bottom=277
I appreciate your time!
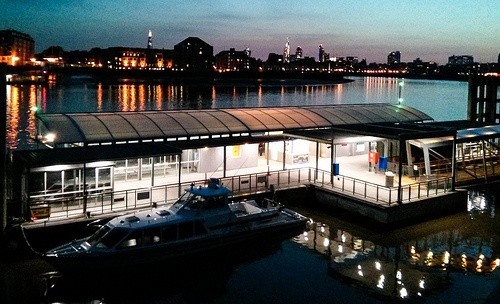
left=41, top=178, right=310, bottom=277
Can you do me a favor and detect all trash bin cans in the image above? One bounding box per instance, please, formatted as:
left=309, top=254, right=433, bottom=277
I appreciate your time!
left=385, top=172, right=394, bottom=188
